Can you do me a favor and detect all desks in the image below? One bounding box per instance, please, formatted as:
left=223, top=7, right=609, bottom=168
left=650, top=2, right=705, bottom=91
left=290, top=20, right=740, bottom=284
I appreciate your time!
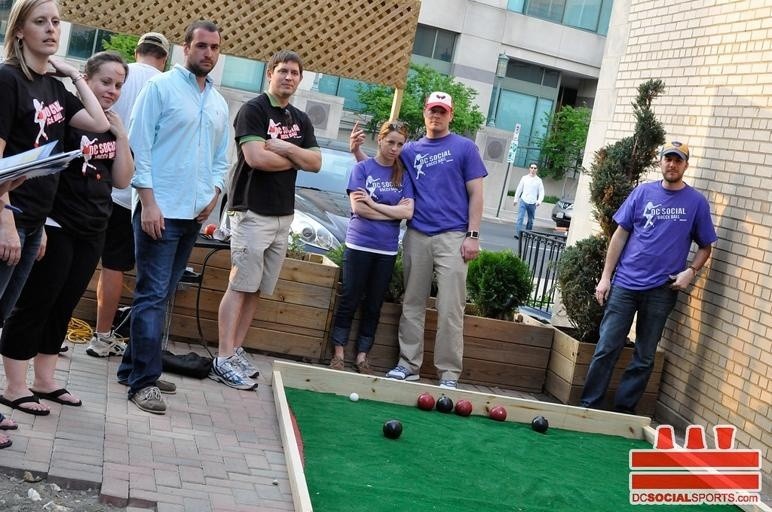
left=159, top=234, right=230, bottom=359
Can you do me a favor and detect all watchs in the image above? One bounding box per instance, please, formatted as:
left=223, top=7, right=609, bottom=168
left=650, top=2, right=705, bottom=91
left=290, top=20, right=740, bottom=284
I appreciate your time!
left=464, top=229, right=481, bottom=239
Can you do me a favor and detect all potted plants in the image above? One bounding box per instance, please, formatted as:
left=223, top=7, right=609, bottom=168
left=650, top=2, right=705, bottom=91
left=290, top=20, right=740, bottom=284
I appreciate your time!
left=323, top=245, right=555, bottom=394
left=546, top=77, right=668, bottom=418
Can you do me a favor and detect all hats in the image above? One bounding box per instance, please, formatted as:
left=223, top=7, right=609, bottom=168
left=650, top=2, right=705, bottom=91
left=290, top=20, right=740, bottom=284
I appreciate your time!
left=661, top=142, right=689, bottom=160
left=137, top=32, right=169, bottom=54
left=425, top=91, right=453, bottom=112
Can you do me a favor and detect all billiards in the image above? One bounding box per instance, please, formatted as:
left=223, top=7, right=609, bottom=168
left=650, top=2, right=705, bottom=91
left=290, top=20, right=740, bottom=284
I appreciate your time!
left=204, top=223, right=216, bottom=234
left=532, top=416, right=548, bottom=433
left=490, top=406, right=506, bottom=421
left=350, top=393, right=359, bottom=402
left=383, top=421, right=401, bottom=439
left=417, top=395, right=472, bottom=417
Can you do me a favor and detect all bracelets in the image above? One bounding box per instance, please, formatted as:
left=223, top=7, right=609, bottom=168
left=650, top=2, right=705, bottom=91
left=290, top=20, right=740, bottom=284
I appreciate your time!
left=688, top=263, right=698, bottom=277
left=72, top=72, right=87, bottom=86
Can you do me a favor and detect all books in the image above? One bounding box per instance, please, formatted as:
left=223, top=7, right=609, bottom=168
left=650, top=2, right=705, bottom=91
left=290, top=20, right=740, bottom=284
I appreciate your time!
left=1, top=138, right=83, bottom=185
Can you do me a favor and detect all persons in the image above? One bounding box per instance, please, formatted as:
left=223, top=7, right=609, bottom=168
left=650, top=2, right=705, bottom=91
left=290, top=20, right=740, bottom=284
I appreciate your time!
left=208, top=51, right=322, bottom=389
left=0, top=51, right=133, bottom=417
left=87, top=27, right=175, bottom=359
left=349, top=89, right=488, bottom=389
left=114, top=20, right=237, bottom=416
left=0, top=0, right=111, bottom=448
left=512, top=162, right=547, bottom=239
left=578, top=141, right=720, bottom=413
left=327, top=116, right=416, bottom=372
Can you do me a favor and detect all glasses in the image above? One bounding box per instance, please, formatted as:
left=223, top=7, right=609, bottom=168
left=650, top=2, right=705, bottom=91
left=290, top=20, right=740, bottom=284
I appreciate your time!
left=284, top=109, right=293, bottom=129
left=530, top=167, right=537, bottom=169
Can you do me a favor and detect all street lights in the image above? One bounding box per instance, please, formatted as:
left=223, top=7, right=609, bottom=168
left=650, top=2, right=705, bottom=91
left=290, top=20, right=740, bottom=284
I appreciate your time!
left=488, top=49, right=510, bottom=128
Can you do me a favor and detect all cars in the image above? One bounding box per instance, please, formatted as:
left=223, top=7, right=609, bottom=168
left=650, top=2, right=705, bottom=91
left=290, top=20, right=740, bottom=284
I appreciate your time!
left=551, top=196, right=576, bottom=228
left=220, top=137, right=408, bottom=264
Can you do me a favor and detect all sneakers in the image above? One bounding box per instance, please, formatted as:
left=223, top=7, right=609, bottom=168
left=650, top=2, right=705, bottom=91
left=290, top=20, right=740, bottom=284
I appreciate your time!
left=119, top=379, right=176, bottom=414
left=440, top=378, right=457, bottom=389
left=207, top=347, right=259, bottom=390
left=386, top=366, right=420, bottom=381
left=352, top=360, right=373, bottom=375
left=86, top=330, right=128, bottom=357
left=330, top=356, right=344, bottom=370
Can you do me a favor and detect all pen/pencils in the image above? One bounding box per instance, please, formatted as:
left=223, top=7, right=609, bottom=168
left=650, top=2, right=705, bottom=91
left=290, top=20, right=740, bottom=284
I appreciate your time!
left=4, top=204, right=23, bottom=216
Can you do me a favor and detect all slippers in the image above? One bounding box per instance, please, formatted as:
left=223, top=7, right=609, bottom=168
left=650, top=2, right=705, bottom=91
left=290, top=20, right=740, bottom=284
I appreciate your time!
left=0, top=388, right=81, bottom=449
